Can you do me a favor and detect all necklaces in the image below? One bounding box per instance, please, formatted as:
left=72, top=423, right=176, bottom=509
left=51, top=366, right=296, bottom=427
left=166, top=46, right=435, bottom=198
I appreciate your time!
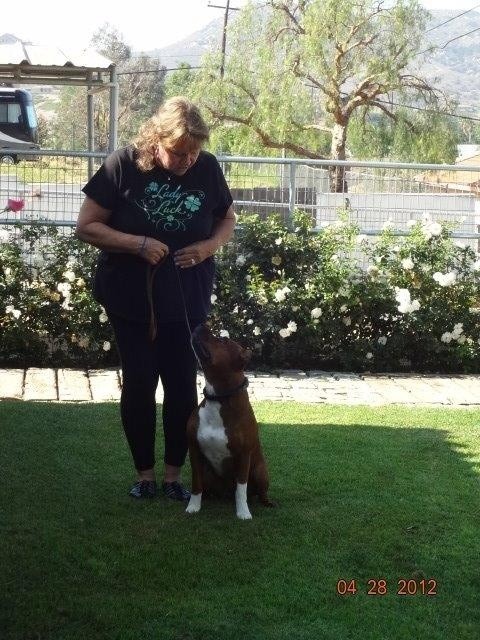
left=167, top=175, right=172, bottom=181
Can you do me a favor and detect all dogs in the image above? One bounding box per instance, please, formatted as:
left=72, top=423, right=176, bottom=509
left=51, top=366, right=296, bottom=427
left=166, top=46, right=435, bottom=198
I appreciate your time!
left=184, top=323, right=278, bottom=520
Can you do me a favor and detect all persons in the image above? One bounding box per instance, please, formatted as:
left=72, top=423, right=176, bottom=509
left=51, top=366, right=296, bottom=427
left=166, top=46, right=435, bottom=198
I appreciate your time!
left=73, top=96, right=237, bottom=502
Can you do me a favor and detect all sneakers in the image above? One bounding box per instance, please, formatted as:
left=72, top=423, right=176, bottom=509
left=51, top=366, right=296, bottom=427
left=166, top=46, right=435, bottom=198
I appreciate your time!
left=162, top=479, right=192, bottom=503
left=129, top=475, right=157, bottom=501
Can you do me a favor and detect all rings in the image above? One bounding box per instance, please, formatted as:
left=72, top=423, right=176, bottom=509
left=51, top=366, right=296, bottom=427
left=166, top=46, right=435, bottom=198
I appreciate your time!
left=190, top=258, right=195, bottom=266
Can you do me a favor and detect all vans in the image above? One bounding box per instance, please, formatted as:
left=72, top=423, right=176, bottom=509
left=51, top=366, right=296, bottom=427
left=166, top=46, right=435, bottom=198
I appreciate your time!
left=0, top=87, right=40, bottom=166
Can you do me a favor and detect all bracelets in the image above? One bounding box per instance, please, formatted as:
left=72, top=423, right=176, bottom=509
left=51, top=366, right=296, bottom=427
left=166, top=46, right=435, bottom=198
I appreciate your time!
left=135, top=236, right=148, bottom=258
